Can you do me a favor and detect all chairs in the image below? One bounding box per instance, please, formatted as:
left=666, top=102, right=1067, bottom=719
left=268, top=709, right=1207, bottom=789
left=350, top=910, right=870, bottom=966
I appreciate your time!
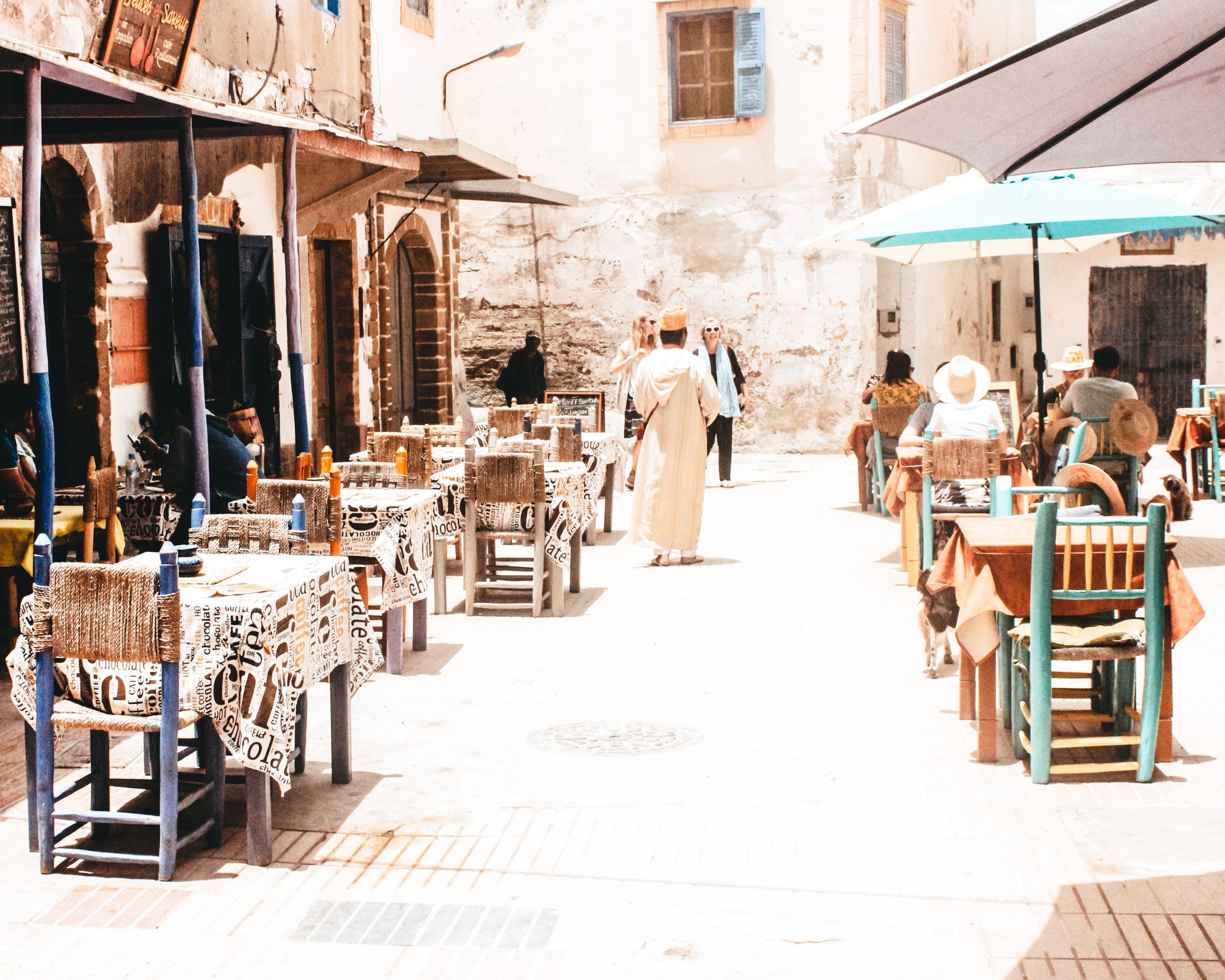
left=856, top=380, right=1224, bottom=784
left=0, top=399, right=623, bottom=881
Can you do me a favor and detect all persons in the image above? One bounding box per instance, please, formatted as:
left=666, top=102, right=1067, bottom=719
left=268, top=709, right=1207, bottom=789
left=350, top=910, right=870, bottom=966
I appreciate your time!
left=634, top=309, right=722, bottom=566
left=691, top=318, right=748, bottom=487
left=0, top=380, right=37, bottom=500
left=503, top=330, right=547, bottom=407
left=133, top=384, right=266, bottom=546
left=847, top=342, right=1136, bottom=510
left=610, top=310, right=658, bottom=489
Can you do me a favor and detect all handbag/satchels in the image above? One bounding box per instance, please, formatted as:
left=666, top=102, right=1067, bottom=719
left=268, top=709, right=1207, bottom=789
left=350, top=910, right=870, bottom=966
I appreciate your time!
left=636, top=422, right=647, bottom=440
left=496, top=368, right=524, bottom=396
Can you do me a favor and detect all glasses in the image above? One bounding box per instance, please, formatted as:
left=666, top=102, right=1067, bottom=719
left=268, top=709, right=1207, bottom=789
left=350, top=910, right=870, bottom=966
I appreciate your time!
left=704, top=326, right=721, bottom=334
left=644, top=320, right=656, bottom=325
left=909, top=367, right=914, bottom=372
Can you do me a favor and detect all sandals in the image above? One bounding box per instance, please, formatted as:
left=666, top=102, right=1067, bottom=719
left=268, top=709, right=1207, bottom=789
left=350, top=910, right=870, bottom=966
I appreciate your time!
left=720, top=480, right=734, bottom=488
left=625, top=470, right=636, bottom=490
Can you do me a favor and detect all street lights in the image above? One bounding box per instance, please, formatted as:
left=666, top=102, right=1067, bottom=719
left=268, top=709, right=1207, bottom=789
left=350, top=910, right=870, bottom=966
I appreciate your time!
left=443, top=42, right=525, bottom=110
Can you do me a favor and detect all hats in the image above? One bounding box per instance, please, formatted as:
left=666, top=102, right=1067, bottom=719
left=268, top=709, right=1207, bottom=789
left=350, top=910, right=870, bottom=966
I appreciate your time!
left=933, top=355, right=991, bottom=404
left=1042, top=416, right=1097, bottom=462
left=1050, top=463, right=1127, bottom=516
left=526, top=330, right=541, bottom=339
left=1050, top=346, right=1094, bottom=372
left=1110, top=399, right=1158, bottom=456
left=659, top=306, right=688, bottom=330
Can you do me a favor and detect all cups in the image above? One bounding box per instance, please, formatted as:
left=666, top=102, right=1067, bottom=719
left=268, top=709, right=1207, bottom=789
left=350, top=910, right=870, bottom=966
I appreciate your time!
left=3, top=493, right=34, bottom=517
left=1046, top=403, right=1059, bottom=427
left=1055, top=398, right=1063, bottom=408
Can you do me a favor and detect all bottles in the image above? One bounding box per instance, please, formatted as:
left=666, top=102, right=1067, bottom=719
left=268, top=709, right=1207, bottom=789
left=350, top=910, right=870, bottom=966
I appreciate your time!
left=175, top=545, right=203, bottom=576
left=124, top=452, right=140, bottom=496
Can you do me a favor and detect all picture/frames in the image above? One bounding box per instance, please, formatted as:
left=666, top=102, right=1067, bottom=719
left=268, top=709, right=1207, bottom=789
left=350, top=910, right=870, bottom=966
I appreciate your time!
left=1121, top=234, right=1175, bottom=255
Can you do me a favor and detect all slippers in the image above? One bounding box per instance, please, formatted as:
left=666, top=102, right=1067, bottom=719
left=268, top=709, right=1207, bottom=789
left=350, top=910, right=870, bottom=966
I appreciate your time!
left=651, top=557, right=669, bottom=567
left=681, top=555, right=704, bottom=565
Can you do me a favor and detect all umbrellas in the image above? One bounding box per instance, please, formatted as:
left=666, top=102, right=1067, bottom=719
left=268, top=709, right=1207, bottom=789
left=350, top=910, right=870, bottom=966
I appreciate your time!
left=806, top=162, right=1225, bottom=486
left=841, top=0, right=1225, bottom=182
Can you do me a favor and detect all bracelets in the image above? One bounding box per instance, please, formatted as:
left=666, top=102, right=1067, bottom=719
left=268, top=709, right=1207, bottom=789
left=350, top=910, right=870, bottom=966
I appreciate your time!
left=627, top=356, right=633, bottom=362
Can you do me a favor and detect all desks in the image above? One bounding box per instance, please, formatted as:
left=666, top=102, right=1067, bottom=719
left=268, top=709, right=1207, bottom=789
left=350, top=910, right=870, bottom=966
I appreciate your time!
left=498, top=431, right=619, bottom=545
left=227, top=485, right=444, bottom=674
left=953, top=515, right=1205, bottom=762
left=430, top=461, right=594, bottom=592
left=54, top=482, right=187, bottom=551
left=5, top=552, right=387, bottom=869
left=0, top=503, right=126, bottom=654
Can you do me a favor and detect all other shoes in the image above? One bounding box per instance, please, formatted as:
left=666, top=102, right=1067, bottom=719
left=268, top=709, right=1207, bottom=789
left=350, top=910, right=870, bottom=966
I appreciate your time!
left=933, top=541, right=939, bottom=561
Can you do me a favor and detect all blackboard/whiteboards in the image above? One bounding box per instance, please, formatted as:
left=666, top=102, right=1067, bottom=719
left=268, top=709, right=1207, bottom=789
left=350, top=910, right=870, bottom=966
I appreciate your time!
left=544, top=390, right=605, bottom=432
left=981, top=381, right=1020, bottom=447
